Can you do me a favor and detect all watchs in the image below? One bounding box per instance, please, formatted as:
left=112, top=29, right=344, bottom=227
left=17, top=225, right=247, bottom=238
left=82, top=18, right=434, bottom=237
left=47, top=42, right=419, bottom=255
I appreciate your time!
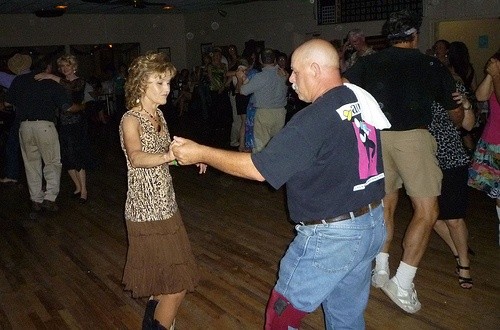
left=463, top=104, right=473, bottom=110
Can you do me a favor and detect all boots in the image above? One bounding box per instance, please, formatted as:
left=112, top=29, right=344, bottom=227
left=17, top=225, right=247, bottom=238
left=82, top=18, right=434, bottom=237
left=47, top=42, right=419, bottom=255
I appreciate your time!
left=149, top=319, right=164, bottom=330
left=264, top=289, right=308, bottom=330
left=142, top=296, right=160, bottom=330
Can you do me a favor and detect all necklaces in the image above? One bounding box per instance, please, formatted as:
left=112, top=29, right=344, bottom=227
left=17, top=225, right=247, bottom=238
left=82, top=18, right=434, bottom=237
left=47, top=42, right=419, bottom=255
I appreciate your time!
left=142, top=108, right=161, bottom=133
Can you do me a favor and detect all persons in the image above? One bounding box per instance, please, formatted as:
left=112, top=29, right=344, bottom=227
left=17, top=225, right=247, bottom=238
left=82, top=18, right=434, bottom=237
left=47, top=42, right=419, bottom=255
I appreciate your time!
left=118, top=51, right=208, bottom=330
left=421, top=50, right=476, bottom=289
left=0, top=28, right=379, bottom=211
left=466, top=49, right=500, bottom=247
left=427, top=40, right=482, bottom=153
left=340, top=10, right=464, bottom=314
left=173, top=38, right=387, bottom=330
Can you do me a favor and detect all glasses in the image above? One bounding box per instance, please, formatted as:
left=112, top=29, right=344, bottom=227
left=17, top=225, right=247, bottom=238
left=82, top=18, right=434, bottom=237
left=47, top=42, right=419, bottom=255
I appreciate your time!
left=279, top=58, right=285, bottom=61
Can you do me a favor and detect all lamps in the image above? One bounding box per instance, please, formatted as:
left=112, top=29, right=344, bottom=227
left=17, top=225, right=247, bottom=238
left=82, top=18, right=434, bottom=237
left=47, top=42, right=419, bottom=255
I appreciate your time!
left=218, top=9, right=227, bottom=17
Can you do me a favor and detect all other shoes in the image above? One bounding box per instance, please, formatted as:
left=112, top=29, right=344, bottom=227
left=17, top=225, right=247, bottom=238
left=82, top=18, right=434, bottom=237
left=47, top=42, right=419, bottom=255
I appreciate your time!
left=0, top=176, right=18, bottom=185
left=72, top=190, right=81, bottom=198
left=79, top=191, right=88, bottom=205
left=42, top=200, right=60, bottom=212
left=29, top=202, right=43, bottom=219
left=239, top=145, right=252, bottom=153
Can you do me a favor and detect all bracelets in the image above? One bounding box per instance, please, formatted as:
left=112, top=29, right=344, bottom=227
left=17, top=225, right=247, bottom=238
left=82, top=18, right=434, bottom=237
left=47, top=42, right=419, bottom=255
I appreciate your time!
left=164, top=154, right=169, bottom=162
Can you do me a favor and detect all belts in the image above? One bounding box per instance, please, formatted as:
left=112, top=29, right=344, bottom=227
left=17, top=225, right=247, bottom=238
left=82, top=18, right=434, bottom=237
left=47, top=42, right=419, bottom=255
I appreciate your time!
left=300, top=200, right=382, bottom=226
left=21, top=119, right=41, bottom=121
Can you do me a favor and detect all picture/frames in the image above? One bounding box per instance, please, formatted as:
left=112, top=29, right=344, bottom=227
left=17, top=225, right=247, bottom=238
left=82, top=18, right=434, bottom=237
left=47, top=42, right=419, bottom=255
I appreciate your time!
left=158, top=47, right=172, bottom=56
left=200, top=42, right=211, bottom=67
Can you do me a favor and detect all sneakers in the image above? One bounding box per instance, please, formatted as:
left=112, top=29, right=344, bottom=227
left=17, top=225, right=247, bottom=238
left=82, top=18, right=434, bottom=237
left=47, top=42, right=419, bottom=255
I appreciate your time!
left=380, top=275, right=422, bottom=316
left=371, top=268, right=391, bottom=288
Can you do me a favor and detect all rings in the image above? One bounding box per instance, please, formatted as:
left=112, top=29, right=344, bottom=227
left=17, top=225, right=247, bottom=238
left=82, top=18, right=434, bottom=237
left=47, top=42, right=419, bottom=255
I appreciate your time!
left=461, top=95, right=463, bottom=98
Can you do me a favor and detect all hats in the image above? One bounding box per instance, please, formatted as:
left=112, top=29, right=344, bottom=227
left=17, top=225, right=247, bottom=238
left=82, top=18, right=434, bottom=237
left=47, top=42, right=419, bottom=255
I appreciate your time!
left=383, top=10, right=422, bottom=40
left=8, top=53, right=32, bottom=76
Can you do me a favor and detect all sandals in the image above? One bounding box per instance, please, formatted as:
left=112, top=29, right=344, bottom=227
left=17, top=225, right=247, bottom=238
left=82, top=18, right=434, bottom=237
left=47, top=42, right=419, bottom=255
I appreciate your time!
left=458, top=265, right=472, bottom=289
left=455, top=255, right=460, bottom=275
